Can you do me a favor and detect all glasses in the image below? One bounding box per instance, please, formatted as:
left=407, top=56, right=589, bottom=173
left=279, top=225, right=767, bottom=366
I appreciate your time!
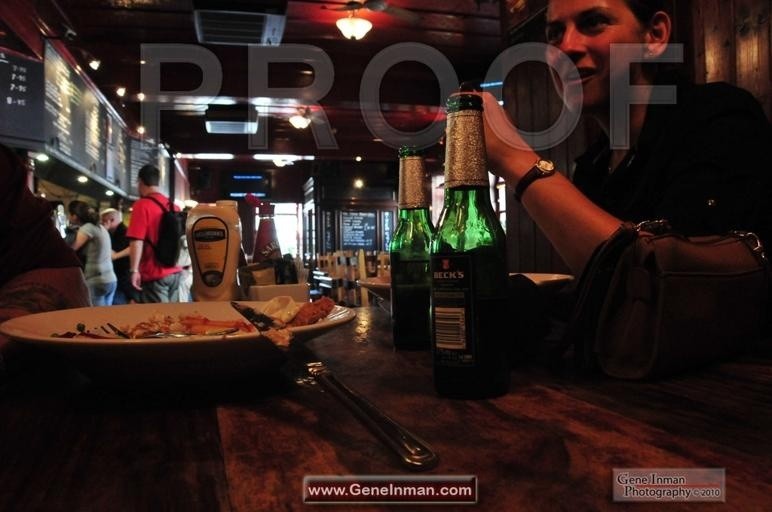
left=336, top=10, right=374, bottom=41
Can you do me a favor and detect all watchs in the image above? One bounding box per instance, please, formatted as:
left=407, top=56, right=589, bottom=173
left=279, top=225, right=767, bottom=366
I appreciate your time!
left=513, top=159, right=556, bottom=203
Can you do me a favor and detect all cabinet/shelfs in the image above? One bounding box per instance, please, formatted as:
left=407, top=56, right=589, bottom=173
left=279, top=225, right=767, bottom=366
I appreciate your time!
left=428, top=91, right=508, bottom=399
left=390, top=145, right=439, bottom=350
left=253, top=198, right=282, bottom=265
left=186, top=204, right=240, bottom=304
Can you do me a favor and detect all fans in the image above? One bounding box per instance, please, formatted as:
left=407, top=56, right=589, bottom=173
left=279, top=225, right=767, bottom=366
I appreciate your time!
left=331, top=0, right=422, bottom=25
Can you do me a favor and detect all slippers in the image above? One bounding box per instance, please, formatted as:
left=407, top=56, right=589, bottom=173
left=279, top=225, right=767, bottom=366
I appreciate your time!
left=141, top=195, right=192, bottom=267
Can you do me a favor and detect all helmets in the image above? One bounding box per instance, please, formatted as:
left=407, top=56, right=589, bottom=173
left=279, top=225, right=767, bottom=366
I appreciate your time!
left=0, top=300, right=357, bottom=350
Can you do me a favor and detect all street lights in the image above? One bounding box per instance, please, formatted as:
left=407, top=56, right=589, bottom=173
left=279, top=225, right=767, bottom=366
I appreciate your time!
left=570, top=224, right=769, bottom=379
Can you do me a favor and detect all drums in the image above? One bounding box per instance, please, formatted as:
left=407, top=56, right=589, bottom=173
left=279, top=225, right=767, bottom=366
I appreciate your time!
left=229, top=300, right=441, bottom=474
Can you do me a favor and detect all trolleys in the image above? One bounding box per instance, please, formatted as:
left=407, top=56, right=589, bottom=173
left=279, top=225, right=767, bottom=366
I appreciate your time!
left=331, top=0, right=422, bottom=25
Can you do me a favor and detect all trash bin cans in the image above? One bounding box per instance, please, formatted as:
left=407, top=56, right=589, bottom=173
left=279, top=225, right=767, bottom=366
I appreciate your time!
left=513, top=159, right=556, bottom=203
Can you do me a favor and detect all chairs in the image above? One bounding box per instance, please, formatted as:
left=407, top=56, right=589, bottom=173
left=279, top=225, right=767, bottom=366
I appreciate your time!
left=316, top=248, right=390, bottom=307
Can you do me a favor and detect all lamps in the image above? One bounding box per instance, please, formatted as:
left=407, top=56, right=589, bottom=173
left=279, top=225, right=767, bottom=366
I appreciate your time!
left=336, top=10, right=374, bottom=41
left=316, top=248, right=390, bottom=307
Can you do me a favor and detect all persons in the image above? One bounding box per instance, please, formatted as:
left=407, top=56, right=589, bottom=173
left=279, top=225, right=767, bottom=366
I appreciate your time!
left=0, top=142, right=194, bottom=385
left=481, top=0, right=772, bottom=287
